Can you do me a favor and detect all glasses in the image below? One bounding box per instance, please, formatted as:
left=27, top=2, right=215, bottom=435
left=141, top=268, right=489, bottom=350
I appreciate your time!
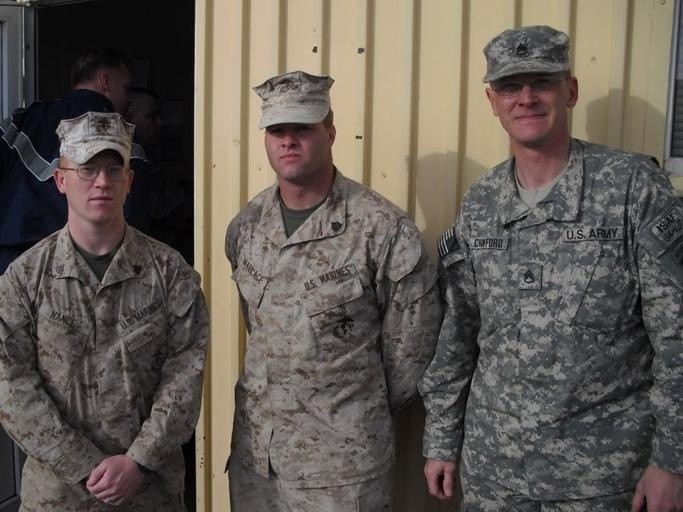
left=58, top=164, right=127, bottom=183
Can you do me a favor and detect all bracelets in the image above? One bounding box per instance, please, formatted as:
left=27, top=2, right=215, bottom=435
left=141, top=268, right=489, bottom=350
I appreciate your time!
left=133, top=461, right=151, bottom=475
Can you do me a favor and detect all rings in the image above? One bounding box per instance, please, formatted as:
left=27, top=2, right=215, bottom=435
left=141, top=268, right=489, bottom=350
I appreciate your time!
left=106, top=496, right=113, bottom=503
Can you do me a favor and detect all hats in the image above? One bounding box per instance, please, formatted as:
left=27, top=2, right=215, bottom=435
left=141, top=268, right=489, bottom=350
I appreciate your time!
left=482, top=26, right=570, bottom=85
left=54, top=112, right=132, bottom=169
left=251, top=71, right=334, bottom=130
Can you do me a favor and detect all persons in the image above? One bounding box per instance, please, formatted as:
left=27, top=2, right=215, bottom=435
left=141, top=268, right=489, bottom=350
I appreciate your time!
left=222, top=69, right=445, bottom=510
left=416, top=25, right=682, bottom=511
left=0, top=110, right=209, bottom=510
left=0, top=49, right=149, bottom=283
left=122, top=84, right=167, bottom=152
